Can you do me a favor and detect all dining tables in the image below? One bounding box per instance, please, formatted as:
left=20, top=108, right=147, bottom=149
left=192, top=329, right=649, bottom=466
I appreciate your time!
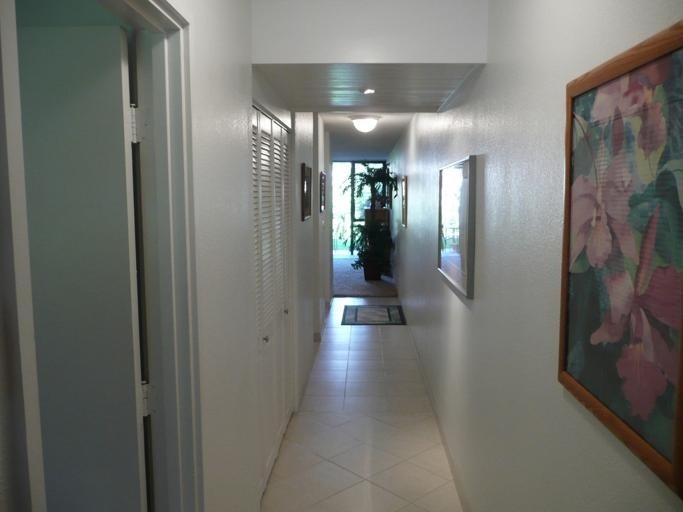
left=343, top=224, right=395, bottom=280
left=341, top=160, right=398, bottom=239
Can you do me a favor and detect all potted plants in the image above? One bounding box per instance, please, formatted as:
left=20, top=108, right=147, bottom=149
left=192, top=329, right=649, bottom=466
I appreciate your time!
left=301, top=163, right=312, bottom=221
left=434, top=155, right=476, bottom=300
left=320, top=171, right=327, bottom=213
left=401, top=175, right=407, bottom=228
left=557, top=20, right=683, bottom=502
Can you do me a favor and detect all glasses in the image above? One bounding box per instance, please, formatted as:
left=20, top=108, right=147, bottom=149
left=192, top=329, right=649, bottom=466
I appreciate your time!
left=347, top=115, right=382, bottom=133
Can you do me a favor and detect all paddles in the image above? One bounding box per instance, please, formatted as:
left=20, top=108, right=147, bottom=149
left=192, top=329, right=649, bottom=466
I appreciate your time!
left=332, top=258, right=398, bottom=297
left=341, top=305, right=408, bottom=325
